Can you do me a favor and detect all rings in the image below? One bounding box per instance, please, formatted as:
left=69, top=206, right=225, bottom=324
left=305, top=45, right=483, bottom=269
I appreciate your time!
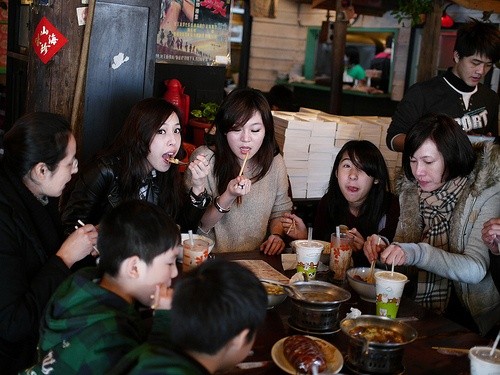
left=237, top=183, right=245, bottom=189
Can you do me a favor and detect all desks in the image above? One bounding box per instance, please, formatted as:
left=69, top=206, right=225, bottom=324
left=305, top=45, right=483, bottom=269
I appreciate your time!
left=277, top=80, right=390, bottom=117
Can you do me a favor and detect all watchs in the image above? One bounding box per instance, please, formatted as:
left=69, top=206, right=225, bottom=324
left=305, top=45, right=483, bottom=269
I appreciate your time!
left=191, top=187, right=207, bottom=201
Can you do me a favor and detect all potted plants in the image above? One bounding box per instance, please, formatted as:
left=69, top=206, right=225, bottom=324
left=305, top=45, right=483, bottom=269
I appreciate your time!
left=189, top=102, right=220, bottom=148
left=391, top=0, right=434, bottom=28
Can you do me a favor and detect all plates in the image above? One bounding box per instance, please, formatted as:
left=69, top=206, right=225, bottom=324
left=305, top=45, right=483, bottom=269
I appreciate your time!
left=271, top=335, right=343, bottom=375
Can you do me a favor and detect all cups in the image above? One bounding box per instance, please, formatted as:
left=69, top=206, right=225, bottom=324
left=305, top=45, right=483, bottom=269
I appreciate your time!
left=294, top=241, right=323, bottom=280
left=469, top=347, right=500, bottom=375
left=330, top=233, right=355, bottom=283
left=374, top=272, right=407, bottom=319
left=184, top=240, right=209, bottom=271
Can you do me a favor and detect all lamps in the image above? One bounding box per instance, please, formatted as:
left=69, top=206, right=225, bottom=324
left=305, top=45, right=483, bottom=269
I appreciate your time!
left=440, top=2, right=456, bottom=28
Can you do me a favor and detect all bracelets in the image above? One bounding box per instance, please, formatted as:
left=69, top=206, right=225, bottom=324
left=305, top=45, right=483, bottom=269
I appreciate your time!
left=273, top=233, right=282, bottom=238
left=214, top=196, right=230, bottom=213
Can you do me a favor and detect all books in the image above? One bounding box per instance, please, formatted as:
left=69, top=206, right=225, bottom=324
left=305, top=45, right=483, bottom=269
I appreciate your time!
left=272, top=107, right=403, bottom=198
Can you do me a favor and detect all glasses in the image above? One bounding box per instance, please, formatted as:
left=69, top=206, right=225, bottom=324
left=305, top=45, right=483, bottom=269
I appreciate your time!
left=57, top=159, right=78, bottom=168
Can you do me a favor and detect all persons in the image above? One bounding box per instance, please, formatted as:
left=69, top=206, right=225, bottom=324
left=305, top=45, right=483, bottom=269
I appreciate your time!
left=17, top=200, right=181, bottom=375
left=0, top=112, right=99, bottom=375
left=280, top=140, right=400, bottom=267
left=108, top=260, right=267, bottom=375
left=185, top=86, right=293, bottom=256
left=73, top=97, right=210, bottom=274
left=386, top=21, right=500, bottom=153
left=160, top=29, right=202, bottom=55
left=343, top=45, right=366, bottom=86
left=371, top=35, right=393, bottom=95
left=363, top=114, right=500, bottom=336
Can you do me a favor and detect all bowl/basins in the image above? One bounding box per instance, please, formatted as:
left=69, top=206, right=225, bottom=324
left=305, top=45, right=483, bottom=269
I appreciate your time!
left=341, top=315, right=418, bottom=347
left=259, top=279, right=288, bottom=309
left=347, top=268, right=385, bottom=303
left=287, top=281, right=352, bottom=310
left=292, top=241, right=330, bottom=263
left=179, top=234, right=214, bottom=259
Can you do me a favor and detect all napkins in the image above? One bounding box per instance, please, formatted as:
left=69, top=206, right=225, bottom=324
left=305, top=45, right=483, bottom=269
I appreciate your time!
left=281, top=254, right=331, bottom=271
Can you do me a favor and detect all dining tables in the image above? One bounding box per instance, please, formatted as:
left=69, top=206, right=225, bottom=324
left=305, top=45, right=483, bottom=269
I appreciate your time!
left=173, top=250, right=500, bottom=375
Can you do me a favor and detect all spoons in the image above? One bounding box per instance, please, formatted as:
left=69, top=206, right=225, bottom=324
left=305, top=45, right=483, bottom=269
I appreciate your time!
left=167, top=159, right=198, bottom=165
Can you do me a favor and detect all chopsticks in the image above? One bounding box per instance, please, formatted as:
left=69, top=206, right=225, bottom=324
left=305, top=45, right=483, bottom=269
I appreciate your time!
left=75, top=220, right=99, bottom=255
left=286, top=218, right=297, bottom=234
left=433, top=347, right=469, bottom=351
left=369, top=237, right=380, bottom=271
left=234, top=153, right=249, bottom=189
